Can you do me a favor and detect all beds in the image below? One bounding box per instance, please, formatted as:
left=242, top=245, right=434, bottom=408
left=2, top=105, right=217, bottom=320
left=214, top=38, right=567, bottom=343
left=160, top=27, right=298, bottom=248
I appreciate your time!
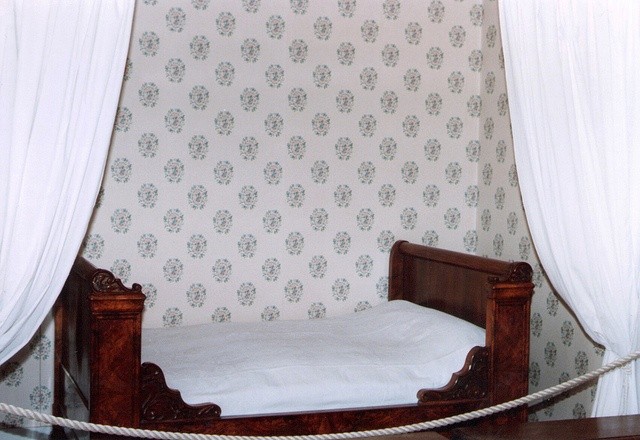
left=52, top=242, right=533, bottom=438
